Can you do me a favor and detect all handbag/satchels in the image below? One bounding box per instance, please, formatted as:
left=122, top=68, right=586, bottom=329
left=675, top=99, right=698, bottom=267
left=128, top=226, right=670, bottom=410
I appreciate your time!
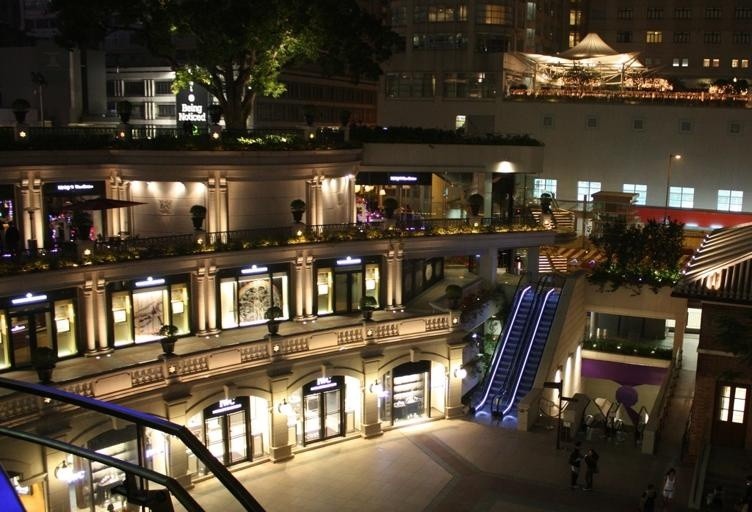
left=570, top=465, right=580, bottom=474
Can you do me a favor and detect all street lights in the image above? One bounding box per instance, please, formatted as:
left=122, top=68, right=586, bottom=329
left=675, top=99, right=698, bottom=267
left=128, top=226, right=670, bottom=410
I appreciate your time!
left=32, top=86, right=45, bottom=127
left=664, top=152, right=682, bottom=225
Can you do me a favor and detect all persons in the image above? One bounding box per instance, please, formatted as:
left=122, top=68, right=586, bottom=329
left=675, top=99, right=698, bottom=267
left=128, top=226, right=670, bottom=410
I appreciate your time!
left=702, top=485, right=726, bottom=511
left=646, top=486, right=658, bottom=512
left=734, top=494, right=750, bottom=511
left=581, top=447, right=598, bottom=495
left=568, top=443, right=583, bottom=488
left=640, top=490, right=649, bottom=511
left=658, top=467, right=680, bottom=511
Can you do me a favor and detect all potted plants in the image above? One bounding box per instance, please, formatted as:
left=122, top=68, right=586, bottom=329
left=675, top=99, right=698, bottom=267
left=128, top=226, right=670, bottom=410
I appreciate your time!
left=469, top=194, right=484, bottom=216
left=71, top=212, right=94, bottom=240
left=35, top=346, right=57, bottom=382
left=540, top=193, right=553, bottom=213
left=289, top=199, right=305, bottom=222
left=190, top=205, right=206, bottom=229
left=359, top=295, right=377, bottom=320
left=444, top=284, right=462, bottom=310
left=264, top=306, right=284, bottom=334
left=158, top=325, right=178, bottom=354
left=383, top=197, right=398, bottom=216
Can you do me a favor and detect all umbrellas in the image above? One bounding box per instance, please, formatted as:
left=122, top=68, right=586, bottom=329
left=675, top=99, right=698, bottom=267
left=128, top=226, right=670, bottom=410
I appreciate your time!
left=60, top=195, right=150, bottom=236
